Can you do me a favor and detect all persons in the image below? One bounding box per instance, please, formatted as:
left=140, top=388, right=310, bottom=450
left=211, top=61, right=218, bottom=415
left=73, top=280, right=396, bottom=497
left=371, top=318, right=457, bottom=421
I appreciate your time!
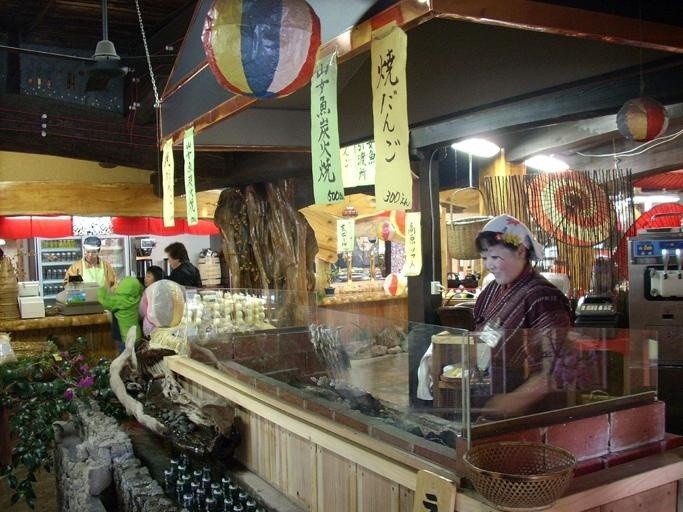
left=474, top=214, right=571, bottom=417
left=138, top=264, right=166, bottom=338
left=164, top=240, right=202, bottom=288
left=96, top=277, right=143, bottom=353
left=63, top=238, right=116, bottom=291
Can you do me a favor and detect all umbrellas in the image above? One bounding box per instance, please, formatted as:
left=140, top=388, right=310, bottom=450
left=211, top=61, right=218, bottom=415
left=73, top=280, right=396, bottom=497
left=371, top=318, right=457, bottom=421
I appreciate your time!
left=525, top=169, right=617, bottom=273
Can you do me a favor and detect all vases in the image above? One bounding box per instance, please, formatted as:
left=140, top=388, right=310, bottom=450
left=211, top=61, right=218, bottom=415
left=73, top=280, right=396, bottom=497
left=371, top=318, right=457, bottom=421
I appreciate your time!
left=63, top=436, right=110, bottom=497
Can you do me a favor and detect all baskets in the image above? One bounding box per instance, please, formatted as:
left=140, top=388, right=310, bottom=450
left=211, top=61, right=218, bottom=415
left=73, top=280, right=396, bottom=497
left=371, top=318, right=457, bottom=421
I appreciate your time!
left=462, top=441, right=579, bottom=507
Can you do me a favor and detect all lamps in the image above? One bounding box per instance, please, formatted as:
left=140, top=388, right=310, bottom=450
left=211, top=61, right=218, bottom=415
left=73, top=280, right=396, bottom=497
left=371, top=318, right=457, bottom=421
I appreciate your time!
left=625, top=191, right=679, bottom=207
left=524, top=153, right=571, bottom=178
left=450, top=135, right=502, bottom=160
left=94, top=5, right=122, bottom=67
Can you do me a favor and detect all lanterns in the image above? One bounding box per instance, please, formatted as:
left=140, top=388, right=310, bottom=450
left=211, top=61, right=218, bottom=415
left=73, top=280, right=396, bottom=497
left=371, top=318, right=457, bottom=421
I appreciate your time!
left=201, top=1, right=321, bottom=101
left=617, top=96, right=669, bottom=141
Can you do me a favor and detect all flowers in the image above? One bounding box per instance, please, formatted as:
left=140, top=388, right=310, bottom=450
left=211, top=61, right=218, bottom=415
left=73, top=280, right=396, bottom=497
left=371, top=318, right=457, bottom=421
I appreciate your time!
left=0, top=337, right=126, bottom=507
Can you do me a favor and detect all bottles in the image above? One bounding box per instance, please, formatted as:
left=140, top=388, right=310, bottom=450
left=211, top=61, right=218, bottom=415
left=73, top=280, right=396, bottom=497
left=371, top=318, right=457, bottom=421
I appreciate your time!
left=56, top=253, right=61, bottom=261
left=44, top=287, right=48, bottom=295
left=57, top=269, right=62, bottom=279
left=47, top=269, right=51, bottom=279
left=53, top=286, right=58, bottom=294
left=67, top=252, right=71, bottom=263
left=51, top=269, right=56, bottom=279
left=48, top=286, right=52, bottom=295
left=42, top=240, right=75, bottom=249
left=63, top=269, right=67, bottom=278
left=163, top=452, right=265, bottom=512
left=61, top=252, right=66, bottom=262
left=59, top=285, right=63, bottom=292
left=72, top=252, right=76, bottom=261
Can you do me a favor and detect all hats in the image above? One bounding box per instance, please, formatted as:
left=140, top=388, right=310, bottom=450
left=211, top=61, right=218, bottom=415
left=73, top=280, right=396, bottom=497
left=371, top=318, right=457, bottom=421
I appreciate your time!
left=480, top=216, right=545, bottom=261
